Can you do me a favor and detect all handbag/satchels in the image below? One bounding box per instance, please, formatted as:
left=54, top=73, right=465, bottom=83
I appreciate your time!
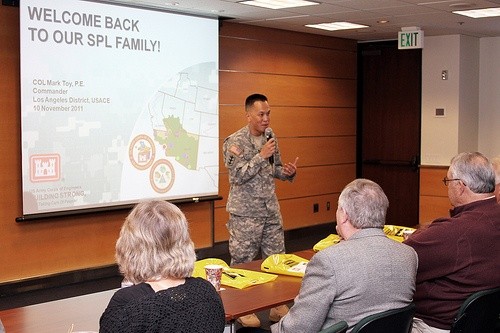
left=190, top=257, right=279, bottom=290
left=383, top=225, right=418, bottom=243
left=313, top=234, right=341, bottom=253
left=261, top=254, right=309, bottom=277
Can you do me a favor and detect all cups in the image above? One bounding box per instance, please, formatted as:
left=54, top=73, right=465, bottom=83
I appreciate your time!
left=403, top=231, right=414, bottom=241
left=205, top=264, right=222, bottom=295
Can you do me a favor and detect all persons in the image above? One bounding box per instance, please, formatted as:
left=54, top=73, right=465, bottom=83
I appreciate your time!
left=235, top=179, right=419, bottom=333
left=222, top=93, right=299, bottom=327
left=98, top=200, right=225, bottom=333
left=402, top=151, right=500, bottom=333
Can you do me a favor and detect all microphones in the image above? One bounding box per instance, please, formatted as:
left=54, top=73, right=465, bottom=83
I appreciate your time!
left=265, top=127, right=274, bottom=166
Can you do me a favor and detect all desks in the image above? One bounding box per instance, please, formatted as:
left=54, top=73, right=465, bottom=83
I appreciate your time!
left=0, top=248, right=314, bottom=333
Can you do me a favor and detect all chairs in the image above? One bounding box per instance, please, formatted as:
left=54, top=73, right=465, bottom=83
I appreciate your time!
left=450, top=287, right=500, bottom=333
left=348, top=302, right=415, bottom=333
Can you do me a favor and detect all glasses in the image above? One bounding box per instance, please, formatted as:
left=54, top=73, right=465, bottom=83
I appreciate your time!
left=442, top=176, right=466, bottom=187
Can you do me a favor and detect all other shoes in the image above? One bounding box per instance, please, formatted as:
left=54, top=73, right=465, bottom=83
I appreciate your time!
left=268, top=304, right=290, bottom=322
left=236, top=313, right=261, bottom=328
